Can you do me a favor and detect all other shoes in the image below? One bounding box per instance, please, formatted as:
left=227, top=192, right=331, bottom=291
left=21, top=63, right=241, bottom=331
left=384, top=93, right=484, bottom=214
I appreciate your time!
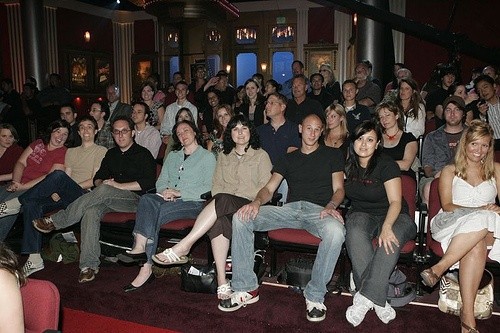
left=0, top=201, right=21, bottom=218
left=21, top=258, right=45, bottom=277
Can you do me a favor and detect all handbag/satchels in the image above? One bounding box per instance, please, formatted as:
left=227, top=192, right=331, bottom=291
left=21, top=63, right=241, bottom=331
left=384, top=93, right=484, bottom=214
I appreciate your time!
left=285, top=256, right=341, bottom=292
left=438, top=263, right=493, bottom=320
left=40, top=230, right=80, bottom=264
left=180, top=263, right=218, bottom=294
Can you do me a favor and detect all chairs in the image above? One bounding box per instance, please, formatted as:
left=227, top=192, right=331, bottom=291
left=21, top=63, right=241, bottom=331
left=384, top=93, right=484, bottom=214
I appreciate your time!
left=0, top=118, right=500, bottom=333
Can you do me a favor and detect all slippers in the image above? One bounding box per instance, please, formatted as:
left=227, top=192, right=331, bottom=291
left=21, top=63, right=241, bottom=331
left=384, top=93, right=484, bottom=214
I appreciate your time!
left=152, top=247, right=190, bottom=264
left=217, top=278, right=233, bottom=299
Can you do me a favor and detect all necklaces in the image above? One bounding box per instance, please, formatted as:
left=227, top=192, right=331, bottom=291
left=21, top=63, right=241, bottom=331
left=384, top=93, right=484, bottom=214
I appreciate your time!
left=385, top=129, right=399, bottom=140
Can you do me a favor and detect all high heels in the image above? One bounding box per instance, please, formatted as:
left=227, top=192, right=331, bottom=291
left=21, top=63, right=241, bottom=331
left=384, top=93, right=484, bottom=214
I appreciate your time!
left=101, top=250, right=148, bottom=266
left=122, top=274, right=155, bottom=293
left=458, top=306, right=480, bottom=333
left=420, top=268, right=439, bottom=287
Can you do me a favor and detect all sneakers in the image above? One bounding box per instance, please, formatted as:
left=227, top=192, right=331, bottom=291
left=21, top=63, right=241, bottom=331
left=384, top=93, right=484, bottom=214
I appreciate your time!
left=32, top=216, right=56, bottom=233
left=346, top=289, right=374, bottom=326
left=305, top=297, right=328, bottom=322
left=78, top=266, right=100, bottom=283
left=374, top=300, right=397, bottom=324
left=217, top=286, right=260, bottom=312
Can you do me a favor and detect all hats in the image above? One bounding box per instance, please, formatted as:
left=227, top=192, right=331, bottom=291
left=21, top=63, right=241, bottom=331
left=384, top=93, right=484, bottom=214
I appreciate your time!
left=443, top=96, right=466, bottom=110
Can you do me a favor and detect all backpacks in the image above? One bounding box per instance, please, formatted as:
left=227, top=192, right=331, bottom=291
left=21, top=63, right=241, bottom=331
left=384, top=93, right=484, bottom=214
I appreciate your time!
left=350, top=265, right=416, bottom=308
left=212, top=248, right=268, bottom=283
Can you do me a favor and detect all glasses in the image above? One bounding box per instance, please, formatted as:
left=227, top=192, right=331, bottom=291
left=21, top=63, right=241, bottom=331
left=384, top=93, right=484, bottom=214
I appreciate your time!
left=88, top=108, right=102, bottom=114
left=265, top=100, right=284, bottom=107
left=113, top=127, right=132, bottom=135
left=319, top=70, right=328, bottom=72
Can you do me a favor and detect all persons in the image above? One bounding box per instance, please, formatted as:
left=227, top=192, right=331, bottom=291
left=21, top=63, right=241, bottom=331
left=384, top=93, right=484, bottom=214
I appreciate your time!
left=0, top=60, right=500, bottom=333
